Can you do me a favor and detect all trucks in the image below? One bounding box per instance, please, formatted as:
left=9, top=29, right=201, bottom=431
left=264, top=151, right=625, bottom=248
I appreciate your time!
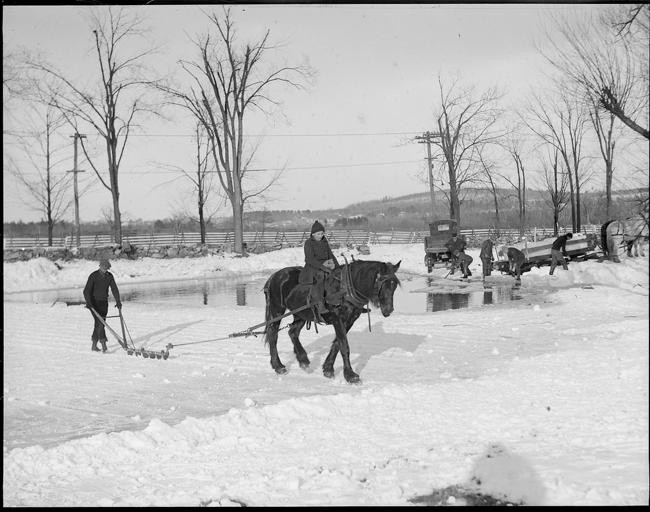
left=422, top=218, right=465, bottom=267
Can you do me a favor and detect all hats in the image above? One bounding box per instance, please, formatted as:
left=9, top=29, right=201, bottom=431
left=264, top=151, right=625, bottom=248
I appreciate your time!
left=311, top=221, right=325, bottom=234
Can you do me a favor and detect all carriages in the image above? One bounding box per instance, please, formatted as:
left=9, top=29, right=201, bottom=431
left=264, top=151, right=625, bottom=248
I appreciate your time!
left=496, top=203, right=649, bottom=275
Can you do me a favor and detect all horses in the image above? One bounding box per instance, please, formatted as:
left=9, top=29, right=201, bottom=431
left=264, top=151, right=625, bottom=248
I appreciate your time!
left=263, top=259, right=403, bottom=386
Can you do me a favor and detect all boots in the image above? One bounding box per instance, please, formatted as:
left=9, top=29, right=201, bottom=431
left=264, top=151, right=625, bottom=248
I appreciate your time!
left=101, top=343, right=107, bottom=352
left=92, top=340, right=100, bottom=351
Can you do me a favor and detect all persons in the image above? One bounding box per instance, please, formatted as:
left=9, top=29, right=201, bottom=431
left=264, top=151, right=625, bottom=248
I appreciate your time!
left=444, top=232, right=468, bottom=275
left=82, top=258, right=123, bottom=352
left=502, top=246, right=525, bottom=280
left=549, top=232, right=573, bottom=275
left=625, top=217, right=645, bottom=257
left=452, top=249, right=474, bottom=278
left=480, top=235, right=497, bottom=278
left=298, top=220, right=342, bottom=317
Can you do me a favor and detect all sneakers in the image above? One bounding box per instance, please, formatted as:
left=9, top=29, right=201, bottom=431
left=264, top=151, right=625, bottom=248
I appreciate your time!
left=319, top=305, right=329, bottom=313
